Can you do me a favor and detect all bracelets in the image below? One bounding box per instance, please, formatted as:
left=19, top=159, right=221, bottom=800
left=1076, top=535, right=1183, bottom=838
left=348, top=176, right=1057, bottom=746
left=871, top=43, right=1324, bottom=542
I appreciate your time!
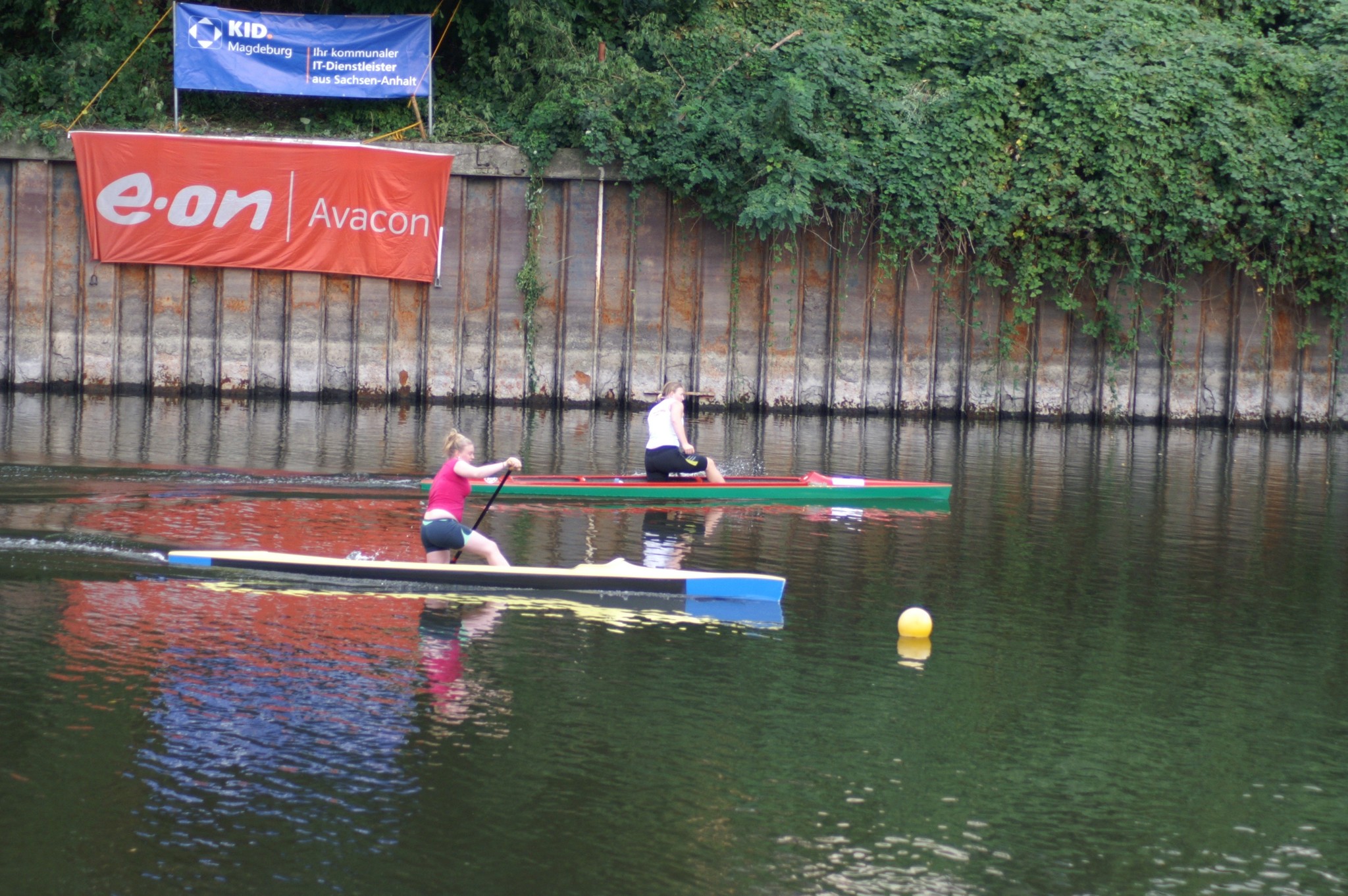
left=503, top=462, right=505, bottom=469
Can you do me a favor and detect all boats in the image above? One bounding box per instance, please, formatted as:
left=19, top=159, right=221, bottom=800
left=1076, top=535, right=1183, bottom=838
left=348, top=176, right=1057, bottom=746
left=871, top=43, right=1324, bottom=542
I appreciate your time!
left=421, top=472, right=953, bottom=499
left=168, top=550, right=787, bottom=603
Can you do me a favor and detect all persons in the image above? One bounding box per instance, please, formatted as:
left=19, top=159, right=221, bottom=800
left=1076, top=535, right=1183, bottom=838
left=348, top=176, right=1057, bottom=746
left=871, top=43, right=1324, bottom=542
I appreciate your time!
left=644, top=382, right=727, bottom=483
left=421, top=428, right=523, bottom=567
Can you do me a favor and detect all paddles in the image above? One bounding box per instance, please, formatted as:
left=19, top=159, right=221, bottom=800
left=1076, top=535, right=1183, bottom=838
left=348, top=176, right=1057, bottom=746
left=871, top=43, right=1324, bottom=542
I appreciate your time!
left=449, top=463, right=521, bottom=564
